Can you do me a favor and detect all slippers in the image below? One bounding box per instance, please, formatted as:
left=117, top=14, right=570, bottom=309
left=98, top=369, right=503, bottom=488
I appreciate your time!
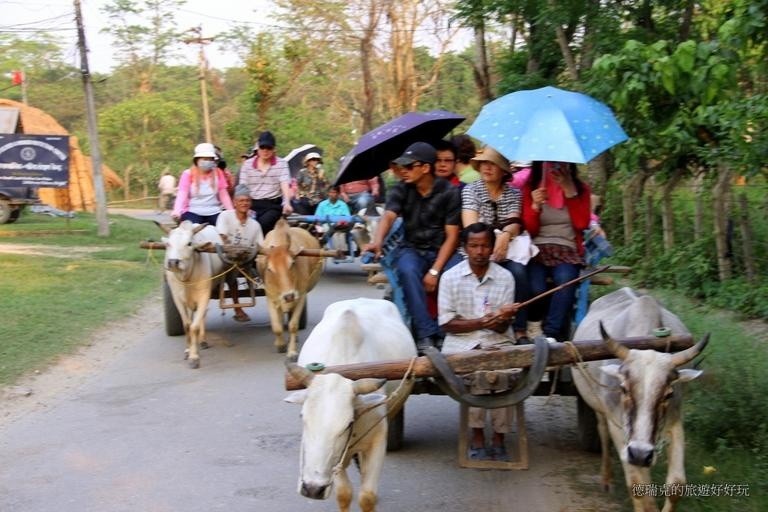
left=469, top=444, right=491, bottom=461
left=233, top=313, right=251, bottom=321
left=492, top=446, right=510, bottom=462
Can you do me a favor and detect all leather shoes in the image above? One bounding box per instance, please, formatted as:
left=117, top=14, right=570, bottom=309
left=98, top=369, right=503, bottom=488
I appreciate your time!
left=517, top=336, right=534, bottom=344
left=417, top=337, right=433, bottom=354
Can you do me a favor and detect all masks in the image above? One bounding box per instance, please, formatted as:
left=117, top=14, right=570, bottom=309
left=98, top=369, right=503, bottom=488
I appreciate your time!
left=198, top=161, right=215, bottom=170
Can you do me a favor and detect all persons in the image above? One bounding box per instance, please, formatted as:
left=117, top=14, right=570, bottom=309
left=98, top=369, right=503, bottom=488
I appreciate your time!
left=436, top=223, right=519, bottom=466
left=160, top=132, right=382, bottom=321
left=361, top=133, right=607, bottom=356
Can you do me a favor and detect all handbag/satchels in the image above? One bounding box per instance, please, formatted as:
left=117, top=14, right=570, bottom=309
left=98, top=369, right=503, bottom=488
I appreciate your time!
left=492, top=229, right=539, bottom=265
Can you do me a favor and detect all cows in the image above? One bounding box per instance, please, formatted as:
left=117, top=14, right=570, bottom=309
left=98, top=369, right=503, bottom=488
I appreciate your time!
left=282, top=298, right=417, bottom=512
left=256, top=218, right=324, bottom=362
left=152, top=220, right=227, bottom=369
left=570, top=285, right=711, bottom=512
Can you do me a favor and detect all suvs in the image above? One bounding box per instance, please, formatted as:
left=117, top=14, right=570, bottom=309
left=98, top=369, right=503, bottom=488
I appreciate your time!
left=0, top=184, right=43, bottom=223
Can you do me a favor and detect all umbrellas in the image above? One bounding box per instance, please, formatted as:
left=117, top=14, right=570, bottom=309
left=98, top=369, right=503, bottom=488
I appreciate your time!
left=462, top=86, right=629, bottom=205
left=330, top=110, right=465, bottom=189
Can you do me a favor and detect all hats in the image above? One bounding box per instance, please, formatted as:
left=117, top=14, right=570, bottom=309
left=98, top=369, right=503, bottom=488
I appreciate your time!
left=304, top=153, right=321, bottom=161
left=471, top=148, right=511, bottom=173
left=259, top=132, right=274, bottom=147
left=193, top=143, right=216, bottom=158
left=393, top=142, right=436, bottom=165
left=511, top=161, right=533, bottom=167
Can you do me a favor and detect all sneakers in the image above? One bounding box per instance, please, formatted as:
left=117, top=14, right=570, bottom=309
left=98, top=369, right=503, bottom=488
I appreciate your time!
left=527, top=321, right=556, bottom=344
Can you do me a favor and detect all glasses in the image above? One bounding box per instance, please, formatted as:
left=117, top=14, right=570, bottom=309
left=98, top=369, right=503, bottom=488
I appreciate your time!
left=405, top=164, right=420, bottom=170
left=435, top=158, right=453, bottom=163
left=260, top=146, right=273, bottom=149
left=491, top=201, right=499, bottom=225
